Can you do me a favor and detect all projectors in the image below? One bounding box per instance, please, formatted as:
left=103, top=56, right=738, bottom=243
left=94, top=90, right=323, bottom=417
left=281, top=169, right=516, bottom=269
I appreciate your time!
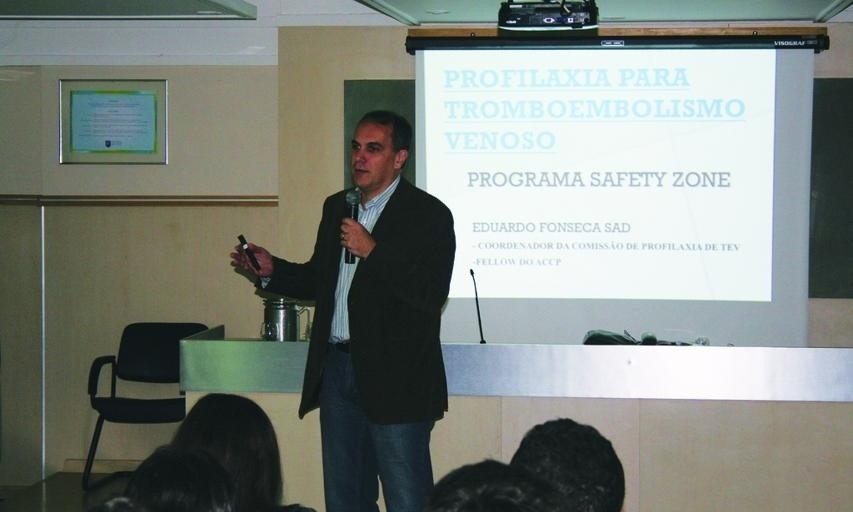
left=497, top=0, right=599, bottom=42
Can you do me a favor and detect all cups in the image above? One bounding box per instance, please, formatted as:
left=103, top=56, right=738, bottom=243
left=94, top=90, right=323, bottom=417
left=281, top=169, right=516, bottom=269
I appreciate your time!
left=259, top=321, right=279, bottom=341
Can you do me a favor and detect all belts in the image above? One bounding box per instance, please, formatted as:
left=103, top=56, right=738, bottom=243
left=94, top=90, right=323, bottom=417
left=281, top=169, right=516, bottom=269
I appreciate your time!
left=327, top=340, right=350, bottom=353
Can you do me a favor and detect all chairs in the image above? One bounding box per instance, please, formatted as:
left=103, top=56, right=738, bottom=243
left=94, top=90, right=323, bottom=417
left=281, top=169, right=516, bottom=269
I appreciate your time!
left=80, top=321, right=210, bottom=486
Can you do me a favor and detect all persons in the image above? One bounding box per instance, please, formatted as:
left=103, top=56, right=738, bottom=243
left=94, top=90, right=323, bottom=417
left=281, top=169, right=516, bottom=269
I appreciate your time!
left=171, top=394, right=318, bottom=512
left=121, top=443, right=237, bottom=512
left=419, top=460, right=558, bottom=511
left=506, top=419, right=626, bottom=512
left=229, top=109, right=456, bottom=512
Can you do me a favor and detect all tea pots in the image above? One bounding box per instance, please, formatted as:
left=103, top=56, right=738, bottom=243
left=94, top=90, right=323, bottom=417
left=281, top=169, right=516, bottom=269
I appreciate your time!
left=261, top=294, right=307, bottom=342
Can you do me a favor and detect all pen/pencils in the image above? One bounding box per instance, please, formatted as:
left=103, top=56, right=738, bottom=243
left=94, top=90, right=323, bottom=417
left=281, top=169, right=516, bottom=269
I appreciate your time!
left=237, top=235, right=264, bottom=276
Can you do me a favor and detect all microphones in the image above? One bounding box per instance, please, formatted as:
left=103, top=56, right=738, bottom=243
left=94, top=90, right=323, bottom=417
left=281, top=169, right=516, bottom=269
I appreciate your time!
left=344, top=189, right=360, bottom=265
left=470, top=269, right=487, bottom=344
